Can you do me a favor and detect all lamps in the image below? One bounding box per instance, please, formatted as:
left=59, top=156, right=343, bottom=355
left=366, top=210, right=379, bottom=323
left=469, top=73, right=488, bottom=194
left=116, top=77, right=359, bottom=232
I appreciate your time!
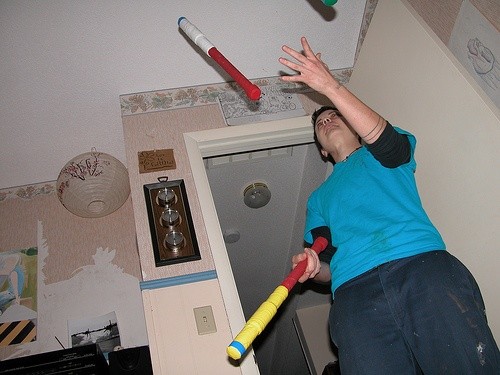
left=243, top=182, right=271, bottom=209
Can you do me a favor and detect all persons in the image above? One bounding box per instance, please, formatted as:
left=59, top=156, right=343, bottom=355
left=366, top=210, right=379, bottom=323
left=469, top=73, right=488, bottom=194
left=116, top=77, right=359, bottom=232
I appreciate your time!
left=278, top=36, right=500, bottom=375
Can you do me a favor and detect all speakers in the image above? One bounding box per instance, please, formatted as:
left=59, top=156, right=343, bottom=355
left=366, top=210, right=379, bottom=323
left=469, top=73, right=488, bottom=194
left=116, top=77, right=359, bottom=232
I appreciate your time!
left=109, top=346, right=153, bottom=375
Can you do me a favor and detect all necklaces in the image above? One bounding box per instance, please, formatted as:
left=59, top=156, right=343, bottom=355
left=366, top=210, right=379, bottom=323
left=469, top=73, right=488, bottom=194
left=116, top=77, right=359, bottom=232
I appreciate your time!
left=342, top=147, right=361, bottom=161
